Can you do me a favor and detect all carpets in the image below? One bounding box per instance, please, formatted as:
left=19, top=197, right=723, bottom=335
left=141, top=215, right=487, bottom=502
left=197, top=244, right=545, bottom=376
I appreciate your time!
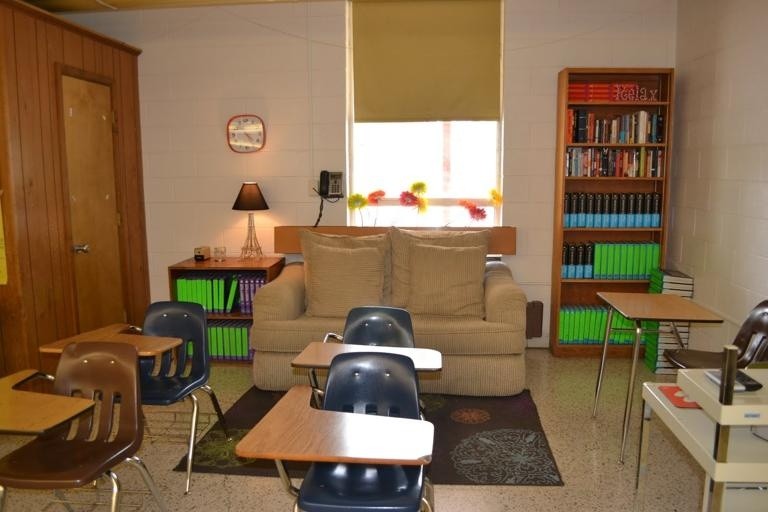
left=171, top=382, right=566, bottom=488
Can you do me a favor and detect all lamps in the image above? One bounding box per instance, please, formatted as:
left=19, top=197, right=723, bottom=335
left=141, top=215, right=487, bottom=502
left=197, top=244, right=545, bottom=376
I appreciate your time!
left=230, top=180, right=271, bottom=257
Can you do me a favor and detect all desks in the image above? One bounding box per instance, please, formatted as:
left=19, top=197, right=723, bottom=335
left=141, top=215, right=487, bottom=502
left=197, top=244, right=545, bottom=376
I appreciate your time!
left=590, top=291, right=725, bottom=468
left=632, top=340, right=768, bottom=512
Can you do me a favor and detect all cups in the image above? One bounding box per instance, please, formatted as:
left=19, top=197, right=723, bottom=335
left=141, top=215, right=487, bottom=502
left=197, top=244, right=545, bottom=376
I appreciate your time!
left=214, top=247, right=225, bottom=262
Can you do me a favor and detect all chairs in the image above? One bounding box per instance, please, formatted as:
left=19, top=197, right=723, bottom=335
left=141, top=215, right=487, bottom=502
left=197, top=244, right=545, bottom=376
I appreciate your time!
left=236, top=352, right=443, bottom=510
left=289, top=304, right=443, bottom=397
left=663, top=296, right=767, bottom=372
left=34, top=303, right=229, bottom=496
left=1, top=340, right=165, bottom=512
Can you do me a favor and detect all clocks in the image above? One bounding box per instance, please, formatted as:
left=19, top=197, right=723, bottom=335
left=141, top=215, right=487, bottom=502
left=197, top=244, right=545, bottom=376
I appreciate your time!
left=228, top=114, right=267, bottom=156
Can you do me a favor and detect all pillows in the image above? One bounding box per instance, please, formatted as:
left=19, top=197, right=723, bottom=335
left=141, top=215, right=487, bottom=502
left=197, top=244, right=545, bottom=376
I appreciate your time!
left=301, top=241, right=385, bottom=321
left=300, top=228, right=392, bottom=302
left=400, top=239, right=486, bottom=316
left=388, top=228, right=493, bottom=309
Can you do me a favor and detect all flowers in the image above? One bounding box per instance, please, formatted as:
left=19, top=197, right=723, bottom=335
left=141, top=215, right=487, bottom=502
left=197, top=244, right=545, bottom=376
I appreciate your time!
left=347, top=180, right=502, bottom=228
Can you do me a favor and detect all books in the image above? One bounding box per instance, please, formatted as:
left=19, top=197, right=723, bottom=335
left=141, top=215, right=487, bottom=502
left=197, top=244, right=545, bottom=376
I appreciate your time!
left=644, top=266, right=696, bottom=376
left=172, top=273, right=267, bottom=362
left=557, top=73, right=660, bottom=345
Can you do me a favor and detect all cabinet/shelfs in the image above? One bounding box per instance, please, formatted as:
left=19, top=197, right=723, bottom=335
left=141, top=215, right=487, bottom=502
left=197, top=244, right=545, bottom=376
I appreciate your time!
left=548, top=65, right=677, bottom=362
left=168, top=254, right=285, bottom=367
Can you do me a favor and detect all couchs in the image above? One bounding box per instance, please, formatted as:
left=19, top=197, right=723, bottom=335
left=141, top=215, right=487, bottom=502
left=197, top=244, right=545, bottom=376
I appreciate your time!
left=246, top=231, right=528, bottom=401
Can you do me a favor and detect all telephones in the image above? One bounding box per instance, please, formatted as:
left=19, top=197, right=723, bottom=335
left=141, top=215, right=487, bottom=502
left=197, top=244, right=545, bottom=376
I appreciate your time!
left=319, top=171, right=344, bottom=199
